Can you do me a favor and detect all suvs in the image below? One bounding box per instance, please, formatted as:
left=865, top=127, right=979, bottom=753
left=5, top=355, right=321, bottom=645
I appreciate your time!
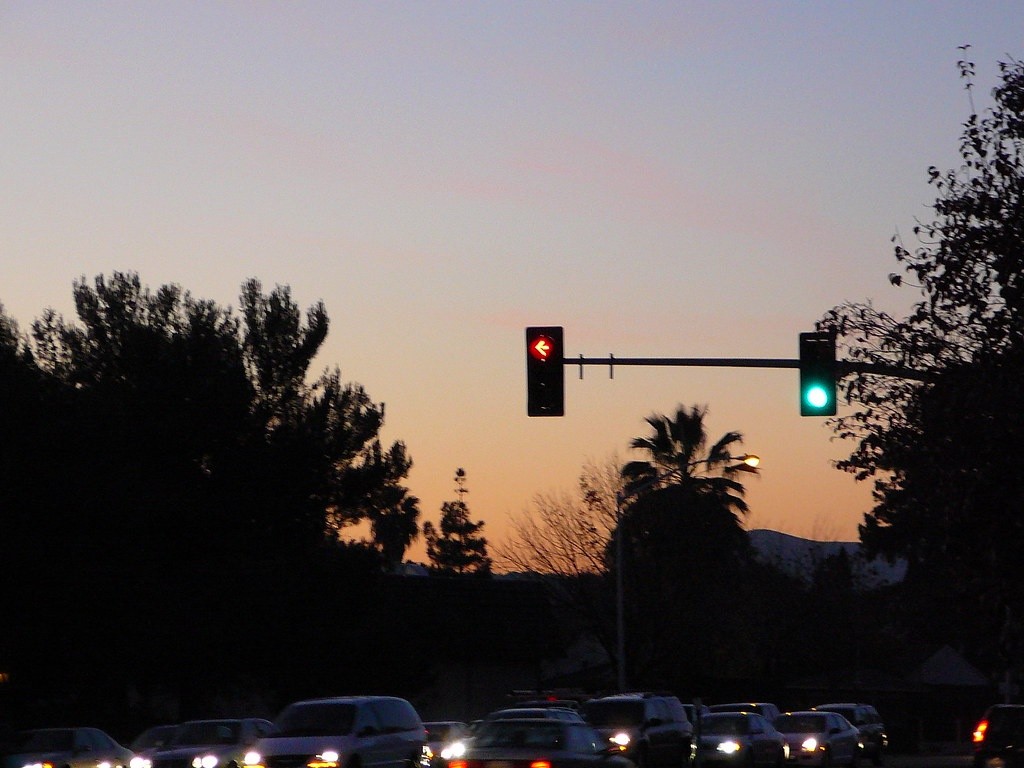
left=583, top=693, right=695, bottom=768
left=973, top=704, right=1024, bottom=768
left=813, top=704, right=888, bottom=768
left=486, top=707, right=582, bottom=721
left=251, top=695, right=428, bottom=768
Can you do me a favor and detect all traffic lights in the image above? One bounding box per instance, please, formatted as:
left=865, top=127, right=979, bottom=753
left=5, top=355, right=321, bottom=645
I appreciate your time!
left=526, top=326, right=564, bottom=417
left=798, top=331, right=838, bottom=418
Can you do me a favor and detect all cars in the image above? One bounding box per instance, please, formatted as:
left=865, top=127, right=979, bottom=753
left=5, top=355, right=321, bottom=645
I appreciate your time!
left=154, top=719, right=281, bottom=768
left=0, top=724, right=136, bottom=768
left=449, top=718, right=634, bottom=768
left=697, top=711, right=790, bottom=768
left=423, top=722, right=467, bottom=768
left=710, top=703, right=780, bottom=714
left=771, top=712, right=859, bottom=768
left=128, top=725, right=178, bottom=754
